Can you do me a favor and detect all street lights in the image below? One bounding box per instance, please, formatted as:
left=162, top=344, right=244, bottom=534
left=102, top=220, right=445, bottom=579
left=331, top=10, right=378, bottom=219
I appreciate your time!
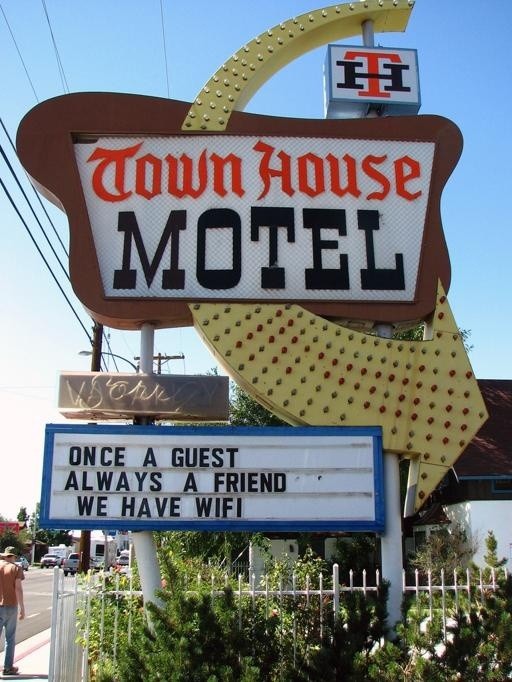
left=79, top=350, right=138, bottom=374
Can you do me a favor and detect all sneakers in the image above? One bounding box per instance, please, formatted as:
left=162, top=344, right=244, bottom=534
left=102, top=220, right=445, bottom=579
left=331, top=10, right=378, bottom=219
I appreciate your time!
left=4, top=666, right=19, bottom=675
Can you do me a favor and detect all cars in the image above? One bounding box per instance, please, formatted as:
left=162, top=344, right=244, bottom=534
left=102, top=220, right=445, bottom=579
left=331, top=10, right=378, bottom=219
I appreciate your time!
left=12, top=535, right=131, bottom=577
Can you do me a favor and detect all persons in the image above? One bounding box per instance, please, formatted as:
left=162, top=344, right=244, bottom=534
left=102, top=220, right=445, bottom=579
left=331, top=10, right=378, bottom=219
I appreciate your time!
left=0, top=546, right=26, bottom=676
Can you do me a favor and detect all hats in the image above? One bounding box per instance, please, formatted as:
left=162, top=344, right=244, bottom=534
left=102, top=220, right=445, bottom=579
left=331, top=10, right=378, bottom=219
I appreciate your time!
left=0, top=546, right=17, bottom=557
